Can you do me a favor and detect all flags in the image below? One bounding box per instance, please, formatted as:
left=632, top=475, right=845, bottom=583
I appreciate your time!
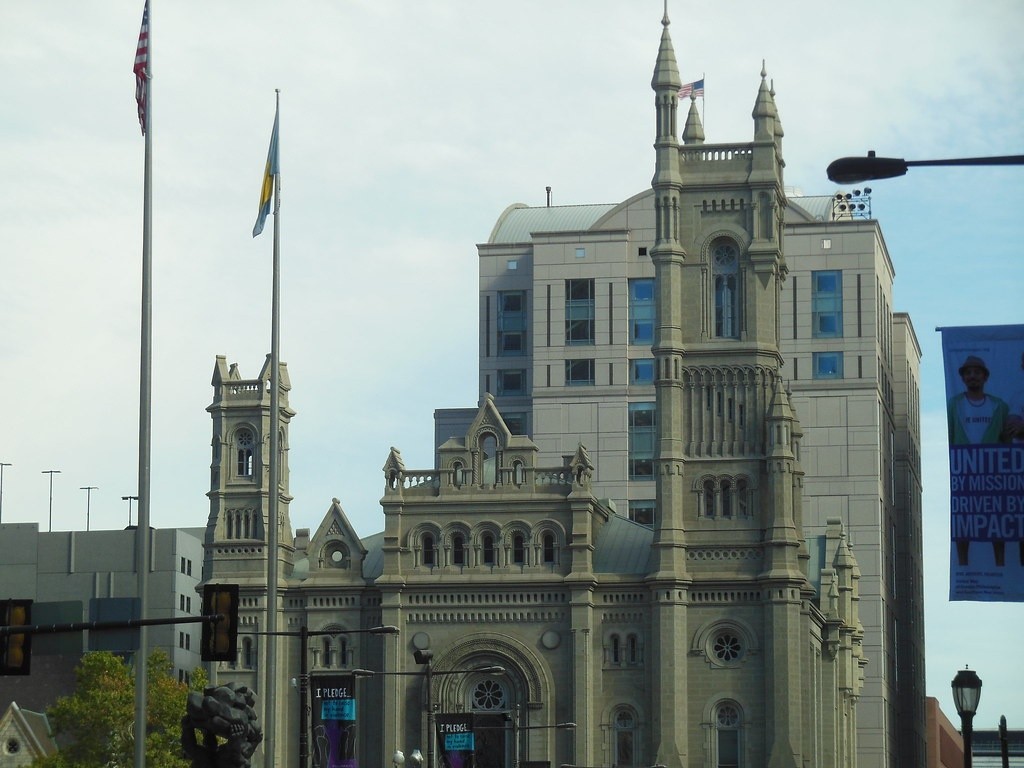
left=678, top=79, right=704, bottom=99
left=253, top=109, right=279, bottom=238
left=133, top=0, right=149, bottom=136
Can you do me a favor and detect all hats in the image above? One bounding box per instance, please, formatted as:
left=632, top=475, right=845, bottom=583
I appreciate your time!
left=959, top=357, right=989, bottom=381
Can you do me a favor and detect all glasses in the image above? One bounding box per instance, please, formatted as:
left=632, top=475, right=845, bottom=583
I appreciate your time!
left=963, top=368, right=984, bottom=373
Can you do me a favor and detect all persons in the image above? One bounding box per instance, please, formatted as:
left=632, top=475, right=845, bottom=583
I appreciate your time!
left=947, top=355, right=1012, bottom=566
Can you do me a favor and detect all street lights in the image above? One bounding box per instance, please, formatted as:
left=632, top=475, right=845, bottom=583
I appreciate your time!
left=79, top=487, right=99, bottom=532
left=299, top=625, right=402, bottom=767
left=41, top=471, right=62, bottom=531
left=952, top=662, right=983, bottom=768
left=0, top=463, right=13, bottom=524
left=514, top=703, right=578, bottom=768
left=350, top=648, right=506, bottom=768
left=121, top=496, right=139, bottom=527
